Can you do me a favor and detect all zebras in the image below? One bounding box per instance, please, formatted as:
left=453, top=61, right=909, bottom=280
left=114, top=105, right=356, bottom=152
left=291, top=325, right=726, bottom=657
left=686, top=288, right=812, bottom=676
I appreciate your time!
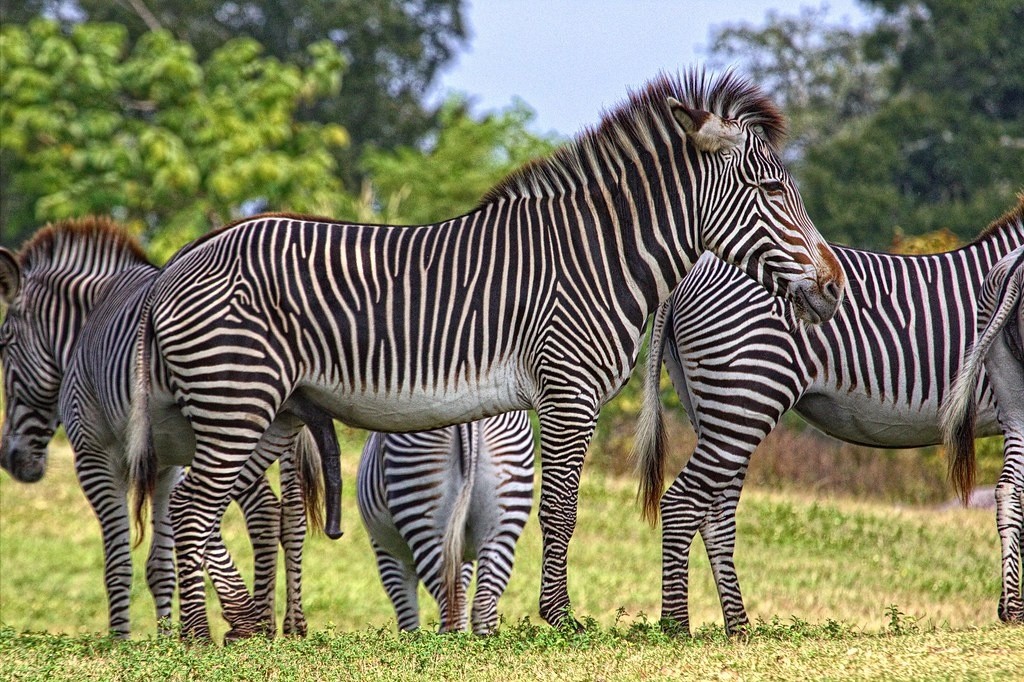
left=1, top=65, right=1024, bottom=645
